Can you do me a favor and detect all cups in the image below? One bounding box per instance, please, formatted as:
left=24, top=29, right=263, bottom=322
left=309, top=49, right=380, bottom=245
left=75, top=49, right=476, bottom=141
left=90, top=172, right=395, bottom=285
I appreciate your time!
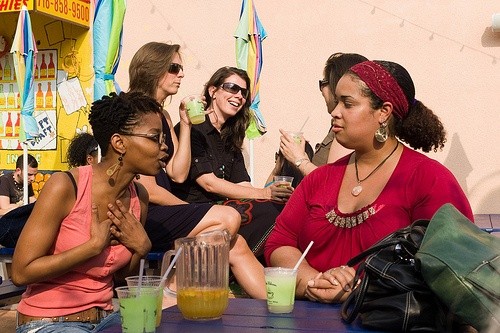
left=181, top=94, right=205, bottom=124
left=125, top=276, right=168, bottom=328
left=115, top=285, right=160, bottom=333
left=284, top=130, right=304, bottom=144
left=263, top=267, right=298, bottom=314
left=273, top=176, right=294, bottom=199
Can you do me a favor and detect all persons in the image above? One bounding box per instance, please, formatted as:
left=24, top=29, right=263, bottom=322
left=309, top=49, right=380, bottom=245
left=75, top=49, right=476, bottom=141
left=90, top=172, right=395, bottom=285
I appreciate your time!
left=11, top=91, right=168, bottom=333
left=66, top=43, right=370, bottom=299
left=232, top=1, right=267, bottom=187
left=0, top=154, right=38, bottom=217
left=264, top=61, right=477, bottom=305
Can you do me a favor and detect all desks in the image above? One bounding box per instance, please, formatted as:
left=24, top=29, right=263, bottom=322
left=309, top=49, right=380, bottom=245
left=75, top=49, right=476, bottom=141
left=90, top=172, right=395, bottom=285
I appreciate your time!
left=97, top=297, right=379, bottom=333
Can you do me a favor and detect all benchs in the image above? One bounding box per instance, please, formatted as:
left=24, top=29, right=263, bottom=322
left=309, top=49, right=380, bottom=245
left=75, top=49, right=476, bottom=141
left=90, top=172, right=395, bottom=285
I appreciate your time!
left=0, top=169, right=63, bottom=199
left=473, top=213, right=500, bottom=239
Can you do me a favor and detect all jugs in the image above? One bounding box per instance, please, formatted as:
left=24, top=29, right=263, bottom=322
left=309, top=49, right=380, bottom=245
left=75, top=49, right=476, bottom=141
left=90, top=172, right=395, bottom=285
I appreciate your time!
left=161, top=228, right=231, bottom=321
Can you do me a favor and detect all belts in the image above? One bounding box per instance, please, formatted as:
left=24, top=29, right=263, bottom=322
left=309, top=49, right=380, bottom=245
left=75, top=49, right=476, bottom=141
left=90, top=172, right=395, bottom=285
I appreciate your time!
left=16, top=307, right=114, bottom=326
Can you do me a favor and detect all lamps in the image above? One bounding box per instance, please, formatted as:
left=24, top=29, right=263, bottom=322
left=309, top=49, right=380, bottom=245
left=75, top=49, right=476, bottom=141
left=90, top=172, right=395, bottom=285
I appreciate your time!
left=488, top=12, right=500, bottom=33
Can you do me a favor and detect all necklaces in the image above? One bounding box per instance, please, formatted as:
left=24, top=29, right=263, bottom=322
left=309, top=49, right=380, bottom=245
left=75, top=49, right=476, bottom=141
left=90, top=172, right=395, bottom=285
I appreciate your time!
left=12, top=175, right=30, bottom=191
left=351, top=140, right=399, bottom=196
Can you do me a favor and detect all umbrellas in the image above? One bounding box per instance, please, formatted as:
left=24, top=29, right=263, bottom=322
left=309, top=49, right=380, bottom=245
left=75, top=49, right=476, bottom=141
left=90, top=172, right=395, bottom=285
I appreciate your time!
left=10, top=5, right=40, bottom=206
left=93, top=0, right=126, bottom=164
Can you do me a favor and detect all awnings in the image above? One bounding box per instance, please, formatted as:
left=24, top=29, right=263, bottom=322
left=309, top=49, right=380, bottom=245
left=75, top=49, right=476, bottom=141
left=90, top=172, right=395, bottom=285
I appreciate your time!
left=0, top=0, right=91, bottom=32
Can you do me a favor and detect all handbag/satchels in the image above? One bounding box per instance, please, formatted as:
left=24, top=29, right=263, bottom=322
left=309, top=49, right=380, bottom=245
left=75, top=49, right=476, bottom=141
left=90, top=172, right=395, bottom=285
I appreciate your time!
left=414, top=202, right=500, bottom=333
left=340, top=218, right=479, bottom=333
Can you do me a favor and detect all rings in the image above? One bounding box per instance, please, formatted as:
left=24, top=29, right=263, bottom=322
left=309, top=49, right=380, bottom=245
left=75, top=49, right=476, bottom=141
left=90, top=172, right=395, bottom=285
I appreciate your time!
left=281, top=192, right=285, bottom=197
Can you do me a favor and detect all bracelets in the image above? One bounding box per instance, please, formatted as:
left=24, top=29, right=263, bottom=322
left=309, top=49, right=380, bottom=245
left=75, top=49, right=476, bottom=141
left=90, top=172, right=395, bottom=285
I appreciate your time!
left=303, top=162, right=310, bottom=176
left=18, top=200, right=23, bottom=206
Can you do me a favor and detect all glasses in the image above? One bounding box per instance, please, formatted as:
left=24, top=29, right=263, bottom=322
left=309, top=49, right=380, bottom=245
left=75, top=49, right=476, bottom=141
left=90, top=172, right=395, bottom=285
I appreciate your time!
left=167, top=63, right=183, bottom=74
left=121, top=132, right=170, bottom=144
left=275, top=152, right=279, bottom=160
left=221, top=82, right=249, bottom=100
left=319, top=79, right=328, bottom=91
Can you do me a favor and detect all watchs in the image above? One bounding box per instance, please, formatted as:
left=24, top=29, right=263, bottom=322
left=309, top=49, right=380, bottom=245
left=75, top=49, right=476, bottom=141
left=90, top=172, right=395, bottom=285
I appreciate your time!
left=295, top=158, right=310, bottom=171
